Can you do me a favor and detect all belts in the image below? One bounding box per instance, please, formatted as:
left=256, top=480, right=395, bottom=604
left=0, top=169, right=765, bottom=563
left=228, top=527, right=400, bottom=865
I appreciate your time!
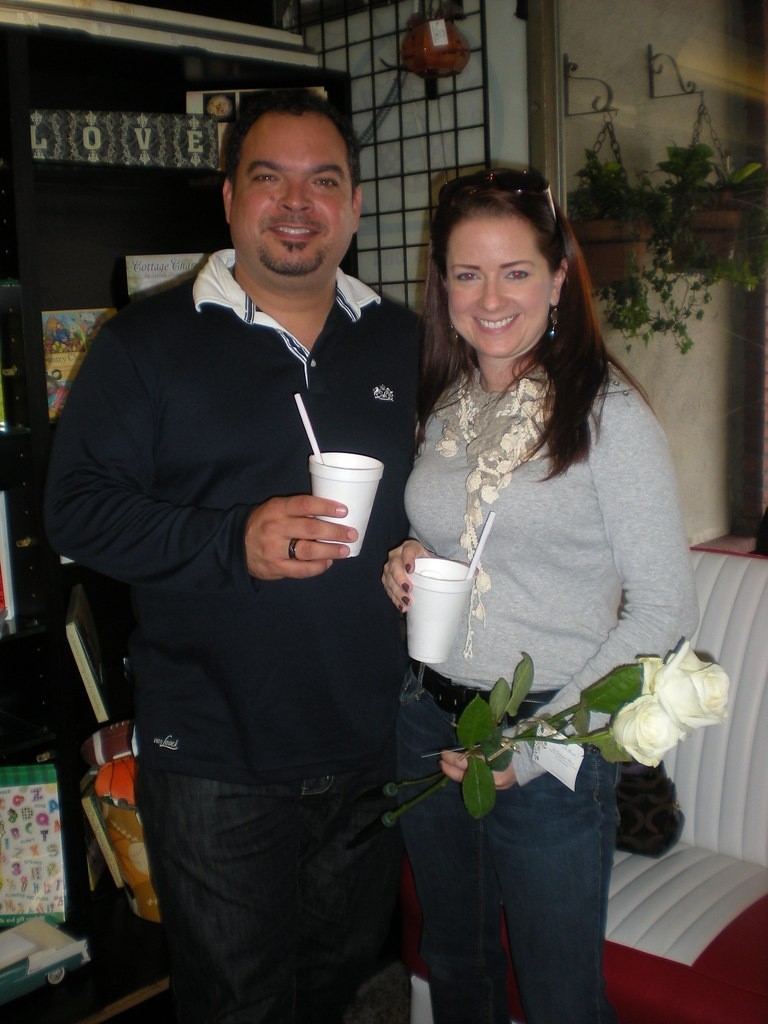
left=410, top=657, right=561, bottom=728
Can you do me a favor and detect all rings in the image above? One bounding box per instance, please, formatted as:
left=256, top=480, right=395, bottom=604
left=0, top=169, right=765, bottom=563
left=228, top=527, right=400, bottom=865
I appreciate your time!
left=288, top=538, right=300, bottom=559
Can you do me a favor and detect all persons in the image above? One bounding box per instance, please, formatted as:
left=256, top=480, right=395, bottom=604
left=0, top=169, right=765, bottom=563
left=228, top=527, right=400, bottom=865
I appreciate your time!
left=46, top=87, right=464, bottom=1023
left=381, top=167, right=700, bottom=1024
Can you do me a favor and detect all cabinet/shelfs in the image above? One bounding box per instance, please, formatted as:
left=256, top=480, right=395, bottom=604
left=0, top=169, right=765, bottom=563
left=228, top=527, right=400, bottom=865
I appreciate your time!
left=0, top=1, right=358, bottom=1023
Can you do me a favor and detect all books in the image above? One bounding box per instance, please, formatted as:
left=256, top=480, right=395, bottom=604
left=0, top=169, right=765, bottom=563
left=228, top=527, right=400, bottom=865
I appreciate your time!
left=126, top=252, right=206, bottom=297
left=42, top=308, right=114, bottom=425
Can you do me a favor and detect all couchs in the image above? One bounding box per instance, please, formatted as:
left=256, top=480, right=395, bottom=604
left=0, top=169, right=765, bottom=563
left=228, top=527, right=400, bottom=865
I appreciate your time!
left=399, top=546, right=768, bottom=1023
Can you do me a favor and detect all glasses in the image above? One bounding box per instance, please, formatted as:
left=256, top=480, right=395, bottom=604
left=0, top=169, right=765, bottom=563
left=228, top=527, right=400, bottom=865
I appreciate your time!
left=438, top=171, right=557, bottom=221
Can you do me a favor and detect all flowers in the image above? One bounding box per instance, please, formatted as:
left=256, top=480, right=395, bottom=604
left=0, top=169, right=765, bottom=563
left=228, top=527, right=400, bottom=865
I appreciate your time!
left=380, top=641, right=730, bottom=828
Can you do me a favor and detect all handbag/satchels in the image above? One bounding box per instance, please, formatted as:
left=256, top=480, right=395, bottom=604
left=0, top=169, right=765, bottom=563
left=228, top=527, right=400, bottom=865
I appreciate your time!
left=615, top=758, right=686, bottom=857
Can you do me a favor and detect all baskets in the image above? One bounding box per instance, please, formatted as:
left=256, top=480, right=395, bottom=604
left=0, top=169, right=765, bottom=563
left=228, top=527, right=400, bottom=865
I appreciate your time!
left=573, top=218, right=645, bottom=284
left=683, top=210, right=742, bottom=263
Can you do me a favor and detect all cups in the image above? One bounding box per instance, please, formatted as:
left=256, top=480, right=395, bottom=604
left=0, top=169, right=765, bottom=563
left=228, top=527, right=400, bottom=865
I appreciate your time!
left=309, top=452, right=384, bottom=558
left=407, top=557, right=479, bottom=663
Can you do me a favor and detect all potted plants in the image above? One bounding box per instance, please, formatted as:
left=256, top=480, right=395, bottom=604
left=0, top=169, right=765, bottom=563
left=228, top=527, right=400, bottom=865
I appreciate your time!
left=566, top=140, right=768, bottom=355
